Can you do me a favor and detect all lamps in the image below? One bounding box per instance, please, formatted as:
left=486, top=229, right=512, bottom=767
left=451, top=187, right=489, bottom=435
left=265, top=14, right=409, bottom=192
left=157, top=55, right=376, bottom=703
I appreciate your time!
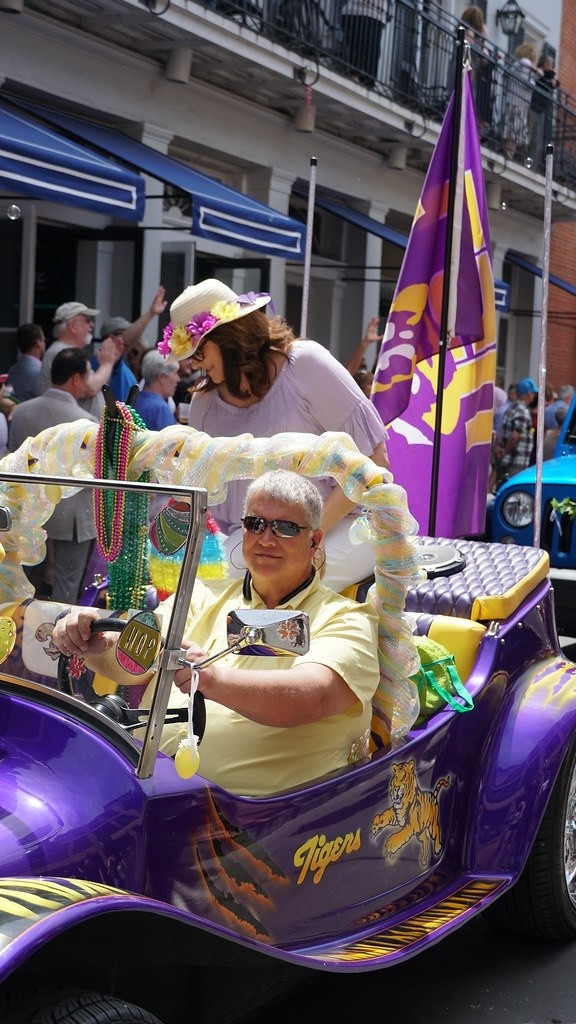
left=387, top=146, right=408, bottom=170
left=485, top=181, right=501, bottom=211
left=0, top=0, right=26, bottom=16
left=496, top=0, right=526, bottom=36
left=292, top=103, right=317, bottom=134
left=163, top=45, right=193, bottom=83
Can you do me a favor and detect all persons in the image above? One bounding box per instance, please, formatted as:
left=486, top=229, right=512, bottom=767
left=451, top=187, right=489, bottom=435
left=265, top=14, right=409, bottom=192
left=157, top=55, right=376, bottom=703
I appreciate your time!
left=520, top=54, right=561, bottom=161
left=338, top=0, right=394, bottom=88
left=499, top=42, right=543, bottom=157
left=0, top=286, right=208, bottom=604
left=446, top=6, right=493, bottom=133
left=254, top=628, right=268, bottom=643
left=347, top=316, right=385, bottom=398
left=51, top=468, right=381, bottom=795
left=291, top=616, right=305, bottom=647
left=158, top=278, right=390, bottom=597
left=487, top=378, right=574, bottom=493
left=270, top=0, right=323, bottom=60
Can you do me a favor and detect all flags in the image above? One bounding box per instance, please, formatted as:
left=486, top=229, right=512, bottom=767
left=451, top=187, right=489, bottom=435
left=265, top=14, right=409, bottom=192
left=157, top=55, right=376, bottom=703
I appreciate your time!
left=371, top=68, right=496, bottom=537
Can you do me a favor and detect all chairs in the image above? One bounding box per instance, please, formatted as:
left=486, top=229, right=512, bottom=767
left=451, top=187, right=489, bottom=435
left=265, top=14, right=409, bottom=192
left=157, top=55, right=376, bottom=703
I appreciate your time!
left=401, top=55, right=449, bottom=122
left=279, top=0, right=349, bottom=72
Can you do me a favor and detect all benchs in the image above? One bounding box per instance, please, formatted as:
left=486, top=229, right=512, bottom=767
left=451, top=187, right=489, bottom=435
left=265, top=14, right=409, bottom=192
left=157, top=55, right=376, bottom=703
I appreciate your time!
left=0, top=600, right=488, bottom=735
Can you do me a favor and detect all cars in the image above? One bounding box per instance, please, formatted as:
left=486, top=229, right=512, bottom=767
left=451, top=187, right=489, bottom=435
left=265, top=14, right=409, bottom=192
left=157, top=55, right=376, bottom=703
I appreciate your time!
left=0, top=419, right=576, bottom=1023
left=488, top=392, right=576, bottom=581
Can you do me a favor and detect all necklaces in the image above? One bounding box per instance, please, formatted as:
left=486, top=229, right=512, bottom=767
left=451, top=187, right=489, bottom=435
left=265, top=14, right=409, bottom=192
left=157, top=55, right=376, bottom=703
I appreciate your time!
left=92, top=400, right=153, bottom=611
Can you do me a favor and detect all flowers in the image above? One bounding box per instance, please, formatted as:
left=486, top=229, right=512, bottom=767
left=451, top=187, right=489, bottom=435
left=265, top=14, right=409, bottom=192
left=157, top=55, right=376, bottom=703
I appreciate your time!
left=156, top=300, right=242, bottom=358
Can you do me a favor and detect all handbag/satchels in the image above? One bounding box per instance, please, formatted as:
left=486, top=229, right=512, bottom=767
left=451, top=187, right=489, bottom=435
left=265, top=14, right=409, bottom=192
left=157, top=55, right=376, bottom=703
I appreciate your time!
left=408, top=635, right=474, bottom=727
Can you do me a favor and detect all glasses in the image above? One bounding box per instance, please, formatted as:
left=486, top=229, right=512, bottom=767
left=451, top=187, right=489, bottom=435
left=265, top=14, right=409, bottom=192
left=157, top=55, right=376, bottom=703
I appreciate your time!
left=240, top=516, right=312, bottom=538
left=186, top=338, right=210, bottom=361
left=130, top=348, right=139, bottom=356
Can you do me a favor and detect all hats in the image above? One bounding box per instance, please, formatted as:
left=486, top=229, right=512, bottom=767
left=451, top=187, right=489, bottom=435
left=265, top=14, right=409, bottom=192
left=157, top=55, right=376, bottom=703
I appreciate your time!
left=516, top=377, right=539, bottom=394
left=101, top=316, right=133, bottom=338
left=157, top=278, right=276, bottom=363
left=53, top=301, right=101, bottom=323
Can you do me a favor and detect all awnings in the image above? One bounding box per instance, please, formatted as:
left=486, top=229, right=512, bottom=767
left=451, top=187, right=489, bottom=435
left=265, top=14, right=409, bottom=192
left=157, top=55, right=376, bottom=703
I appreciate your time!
left=504, top=251, right=576, bottom=318
left=288, top=181, right=507, bottom=310
left=0, top=111, right=146, bottom=222
left=0, top=90, right=305, bottom=260
